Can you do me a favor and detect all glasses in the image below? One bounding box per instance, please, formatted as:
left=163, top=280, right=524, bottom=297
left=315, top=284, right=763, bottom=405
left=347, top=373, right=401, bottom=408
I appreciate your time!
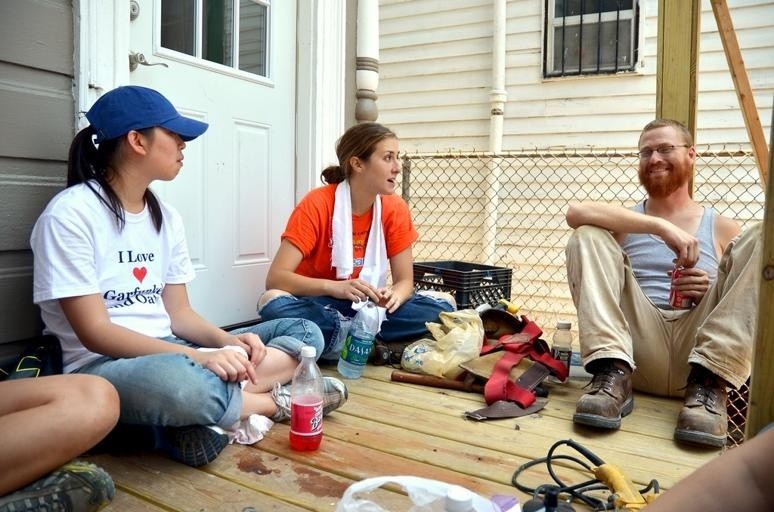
left=637, top=145, right=689, bottom=158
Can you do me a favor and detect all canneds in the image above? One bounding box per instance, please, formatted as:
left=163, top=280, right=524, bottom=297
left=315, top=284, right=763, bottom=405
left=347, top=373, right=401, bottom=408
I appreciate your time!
left=669, top=269, right=693, bottom=312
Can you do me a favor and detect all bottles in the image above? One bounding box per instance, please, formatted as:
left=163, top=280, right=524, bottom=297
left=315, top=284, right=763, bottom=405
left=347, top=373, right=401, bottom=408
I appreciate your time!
left=548, top=322, right=573, bottom=385
left=336, top=298, right=379, bottom=379
left=291, top=347, right=323, bottom=453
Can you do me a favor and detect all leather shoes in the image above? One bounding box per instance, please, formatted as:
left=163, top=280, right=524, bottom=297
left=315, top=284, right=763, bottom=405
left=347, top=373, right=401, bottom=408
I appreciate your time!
left=674, top=376, right=728, bottom=447
left=574, top=361, right=634, bottom=429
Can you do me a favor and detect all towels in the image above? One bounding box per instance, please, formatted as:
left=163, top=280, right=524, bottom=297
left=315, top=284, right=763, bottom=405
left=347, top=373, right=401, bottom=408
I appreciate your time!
left=332, top=178, right=387, bottom=311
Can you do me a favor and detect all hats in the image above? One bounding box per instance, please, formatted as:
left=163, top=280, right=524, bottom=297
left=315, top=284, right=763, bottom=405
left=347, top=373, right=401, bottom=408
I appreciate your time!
left=86, top=85, right=209, bottom=141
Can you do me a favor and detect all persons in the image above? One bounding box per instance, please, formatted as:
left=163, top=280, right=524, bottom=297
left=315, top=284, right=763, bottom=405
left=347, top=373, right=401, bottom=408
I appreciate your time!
left=636, top=421, right=774, bottom=511
left=256, top=123, right=456, bottom=360
left=565, top=119, right=764, bottom=447
left=30, top=83, right=349, bottom=468
left=2, top=372, right=121, bottom=510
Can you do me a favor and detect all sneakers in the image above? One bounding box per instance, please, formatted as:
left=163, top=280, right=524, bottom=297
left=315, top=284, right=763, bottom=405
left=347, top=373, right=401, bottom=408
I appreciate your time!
left=0, top=460, right=116, bottom=512
left=374, top=334, right=436, bottom=367
left=271, top=377, right=348, bottom=425
left=140, top=422, right=228, bottom=468
